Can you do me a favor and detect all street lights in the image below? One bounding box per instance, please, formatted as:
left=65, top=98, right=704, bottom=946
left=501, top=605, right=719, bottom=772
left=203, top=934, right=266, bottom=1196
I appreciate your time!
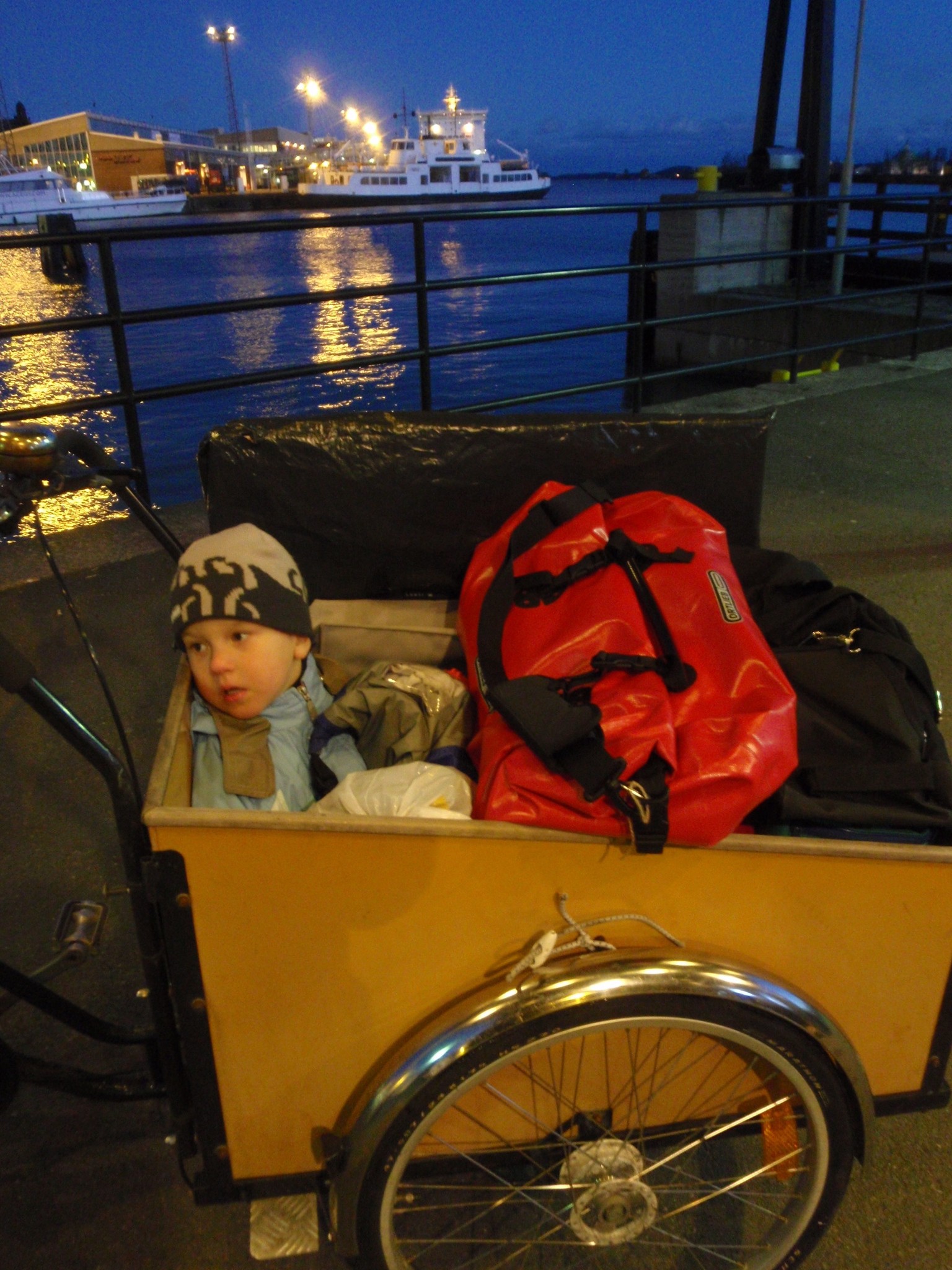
left=340, top=107, right=362, bottom=170
left=79, top=162, right=89, bottom=182
left=205, top=24, right=242, bottom=150
left=295, top=77, right=321, bottom=148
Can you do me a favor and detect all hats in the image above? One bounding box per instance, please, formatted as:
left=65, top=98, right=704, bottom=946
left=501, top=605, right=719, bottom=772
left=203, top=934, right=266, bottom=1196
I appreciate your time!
left=167, top=522, right=317, bottom=645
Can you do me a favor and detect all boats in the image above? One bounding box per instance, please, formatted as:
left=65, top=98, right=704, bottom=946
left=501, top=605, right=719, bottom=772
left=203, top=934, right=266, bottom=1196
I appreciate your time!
left=296, top=79, right=552, bottom=208
left=0, top=166, right=188, bottom=226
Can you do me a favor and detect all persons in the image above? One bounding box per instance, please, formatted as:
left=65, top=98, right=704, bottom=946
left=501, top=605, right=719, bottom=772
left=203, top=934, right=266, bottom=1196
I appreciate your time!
left=171, top=523, right=367, bottom=812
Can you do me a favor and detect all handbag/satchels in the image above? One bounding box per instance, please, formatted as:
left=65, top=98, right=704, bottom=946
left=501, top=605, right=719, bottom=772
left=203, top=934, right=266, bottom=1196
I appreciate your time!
left=445, top=473, right=952, bottom=853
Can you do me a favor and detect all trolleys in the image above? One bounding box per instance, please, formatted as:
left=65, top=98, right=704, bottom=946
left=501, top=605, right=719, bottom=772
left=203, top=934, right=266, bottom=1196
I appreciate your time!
left=0, top=420, right=952, bottom=1270
